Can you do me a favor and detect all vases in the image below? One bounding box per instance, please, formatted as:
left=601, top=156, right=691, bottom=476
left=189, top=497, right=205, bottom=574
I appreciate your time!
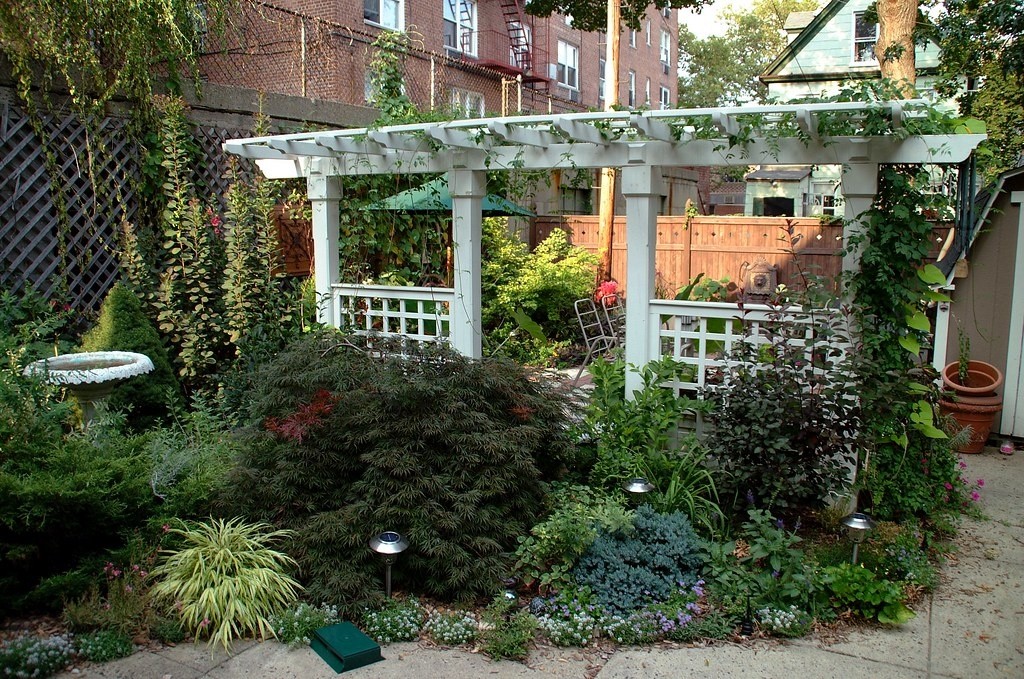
left=938, top=387, right=1003, bottom=455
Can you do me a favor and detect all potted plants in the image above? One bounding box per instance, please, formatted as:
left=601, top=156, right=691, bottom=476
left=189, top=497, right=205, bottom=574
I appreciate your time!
left=940, top=308, right=1004, bottom=399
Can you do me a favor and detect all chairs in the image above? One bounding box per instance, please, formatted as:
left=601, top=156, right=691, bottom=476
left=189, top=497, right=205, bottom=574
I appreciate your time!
left=570, top=294, right=626, bottom=388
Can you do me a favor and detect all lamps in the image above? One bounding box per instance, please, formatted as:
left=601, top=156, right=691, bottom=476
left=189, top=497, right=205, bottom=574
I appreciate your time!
left=839, top=512, right=879, bottom=529
left=369, top=531, right=409, bottom=555
left=622, top=478, right=656, bottom=493
